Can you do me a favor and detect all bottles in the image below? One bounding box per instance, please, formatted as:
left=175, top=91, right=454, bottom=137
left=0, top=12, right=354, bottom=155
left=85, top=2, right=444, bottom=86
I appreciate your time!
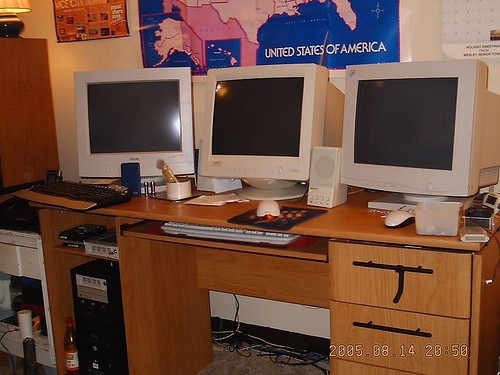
left=64, top=316, right=80, bottom=375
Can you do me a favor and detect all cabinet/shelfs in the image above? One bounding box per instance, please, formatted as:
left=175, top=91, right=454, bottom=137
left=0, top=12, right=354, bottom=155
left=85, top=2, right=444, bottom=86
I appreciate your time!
left=29, top=180, right=500, bottom=375
left=0, top=38, right=61, bottom=187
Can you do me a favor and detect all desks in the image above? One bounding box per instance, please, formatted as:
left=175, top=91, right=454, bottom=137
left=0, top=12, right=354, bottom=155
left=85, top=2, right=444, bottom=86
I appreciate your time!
left=0, top=228, right=57, bottom=368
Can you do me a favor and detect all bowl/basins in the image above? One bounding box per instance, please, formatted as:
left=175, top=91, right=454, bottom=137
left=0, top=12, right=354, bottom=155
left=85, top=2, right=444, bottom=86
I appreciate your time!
left=414, top=201, right=464, bottom=237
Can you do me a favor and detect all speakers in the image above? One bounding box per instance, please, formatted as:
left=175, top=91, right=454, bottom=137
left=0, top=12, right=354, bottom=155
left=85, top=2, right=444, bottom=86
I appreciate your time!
left=414, top=201, right=461, bottom=237
left=307, top=146, right=348, bottom=208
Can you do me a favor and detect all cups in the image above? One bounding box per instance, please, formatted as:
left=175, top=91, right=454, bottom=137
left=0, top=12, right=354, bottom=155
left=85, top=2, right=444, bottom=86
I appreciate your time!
left=166, top=180, right=191, bottom=201
left=18, top=310, right=32, bottom=339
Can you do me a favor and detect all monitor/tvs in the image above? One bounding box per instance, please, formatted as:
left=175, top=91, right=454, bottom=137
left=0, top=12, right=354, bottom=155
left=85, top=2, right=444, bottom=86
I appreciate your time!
left=74, top=66, right=193, bottom=191
left=200, top=63, right=346, bottom=200
left=339, top=57, right=500, bottom=213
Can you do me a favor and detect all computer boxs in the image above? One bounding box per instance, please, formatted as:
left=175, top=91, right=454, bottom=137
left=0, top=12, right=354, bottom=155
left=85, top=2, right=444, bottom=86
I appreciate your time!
left=70, top=258, right=129, bottom=375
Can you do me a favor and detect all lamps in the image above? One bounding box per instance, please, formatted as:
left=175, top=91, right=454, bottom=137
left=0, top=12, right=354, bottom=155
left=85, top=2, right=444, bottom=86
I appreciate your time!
left=0, top=0, right=33, bottom=39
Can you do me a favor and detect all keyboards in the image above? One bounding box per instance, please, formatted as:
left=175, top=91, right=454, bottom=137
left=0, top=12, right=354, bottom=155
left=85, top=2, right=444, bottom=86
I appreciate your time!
left=160, top=221, right=300, bottom=245
left=12, top=179, right=131, bottom=211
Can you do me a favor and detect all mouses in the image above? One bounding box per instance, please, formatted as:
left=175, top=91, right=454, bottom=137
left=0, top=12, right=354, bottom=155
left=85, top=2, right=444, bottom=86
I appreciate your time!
left=383, top=211, right=415, bottom=228
left=256, top=199, right=280, bottom=218
